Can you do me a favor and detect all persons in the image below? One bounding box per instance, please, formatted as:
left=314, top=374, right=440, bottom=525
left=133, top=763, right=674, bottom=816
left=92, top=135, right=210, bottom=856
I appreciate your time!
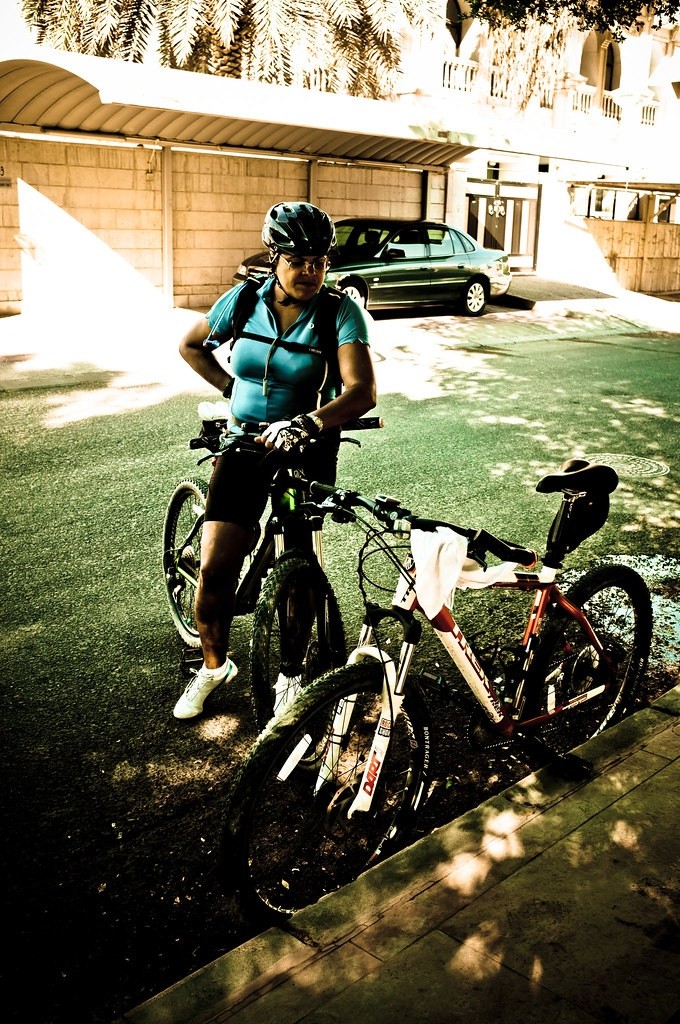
left=171, top=200, right=381, bottom=723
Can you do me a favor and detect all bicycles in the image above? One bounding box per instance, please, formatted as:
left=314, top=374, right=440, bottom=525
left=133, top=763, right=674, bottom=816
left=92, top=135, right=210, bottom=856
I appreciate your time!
left=160, top=410, right=384, bottom=776
left=223, top=458, right=657, bottom=926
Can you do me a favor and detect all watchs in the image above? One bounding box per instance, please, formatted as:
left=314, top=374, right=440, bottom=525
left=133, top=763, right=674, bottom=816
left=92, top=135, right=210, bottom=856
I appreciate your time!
left=306, top=413, right=324, bottom=433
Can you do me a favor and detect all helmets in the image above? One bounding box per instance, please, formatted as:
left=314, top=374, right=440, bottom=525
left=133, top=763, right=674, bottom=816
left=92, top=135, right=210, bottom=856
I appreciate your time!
left=262, top=201, right=339, bottom=258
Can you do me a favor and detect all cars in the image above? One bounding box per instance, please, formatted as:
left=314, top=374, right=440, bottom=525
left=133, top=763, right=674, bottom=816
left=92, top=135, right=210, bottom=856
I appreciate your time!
left=230, top=215, right=513, bottom=317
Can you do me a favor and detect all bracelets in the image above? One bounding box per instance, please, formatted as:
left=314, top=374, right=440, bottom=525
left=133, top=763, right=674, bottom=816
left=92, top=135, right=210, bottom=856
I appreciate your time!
left=223, top=377, right=235, bottom=399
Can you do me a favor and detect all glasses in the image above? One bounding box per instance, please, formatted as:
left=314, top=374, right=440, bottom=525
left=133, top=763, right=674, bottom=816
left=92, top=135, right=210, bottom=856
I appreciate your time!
left=275, top=252, right=334, bottom=272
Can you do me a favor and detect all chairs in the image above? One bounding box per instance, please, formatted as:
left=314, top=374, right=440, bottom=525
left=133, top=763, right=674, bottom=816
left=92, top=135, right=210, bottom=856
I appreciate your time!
left=360, top=230, right=381, bottom=256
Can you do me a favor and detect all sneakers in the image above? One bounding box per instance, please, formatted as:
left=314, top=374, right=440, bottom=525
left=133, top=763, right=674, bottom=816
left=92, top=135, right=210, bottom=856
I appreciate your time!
left=173, top=658, right=238, bottom=721
left=272, top=672, right=304, bottom=728
